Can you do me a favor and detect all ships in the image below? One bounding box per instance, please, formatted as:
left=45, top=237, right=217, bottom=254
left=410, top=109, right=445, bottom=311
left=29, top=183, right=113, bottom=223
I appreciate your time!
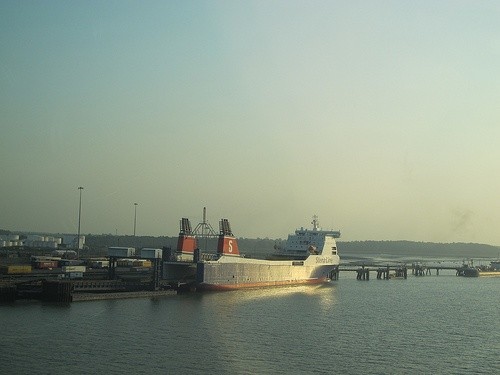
left=161, top=205, right=341, bottom=295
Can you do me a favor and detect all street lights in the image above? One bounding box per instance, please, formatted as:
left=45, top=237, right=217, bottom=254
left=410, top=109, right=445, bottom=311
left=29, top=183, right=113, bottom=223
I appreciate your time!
left=76, top=186, right=84, bottom=257
left=133, top=202, right=138, bottom=255
